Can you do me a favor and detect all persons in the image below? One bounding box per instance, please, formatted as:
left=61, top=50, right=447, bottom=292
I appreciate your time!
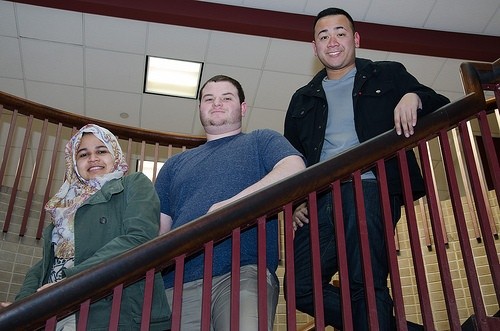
left=0, top=124, right=173, bottom=331
left=154, top=74, right=307, bottom=331
left=283, top=7, right=451, bottom=331
